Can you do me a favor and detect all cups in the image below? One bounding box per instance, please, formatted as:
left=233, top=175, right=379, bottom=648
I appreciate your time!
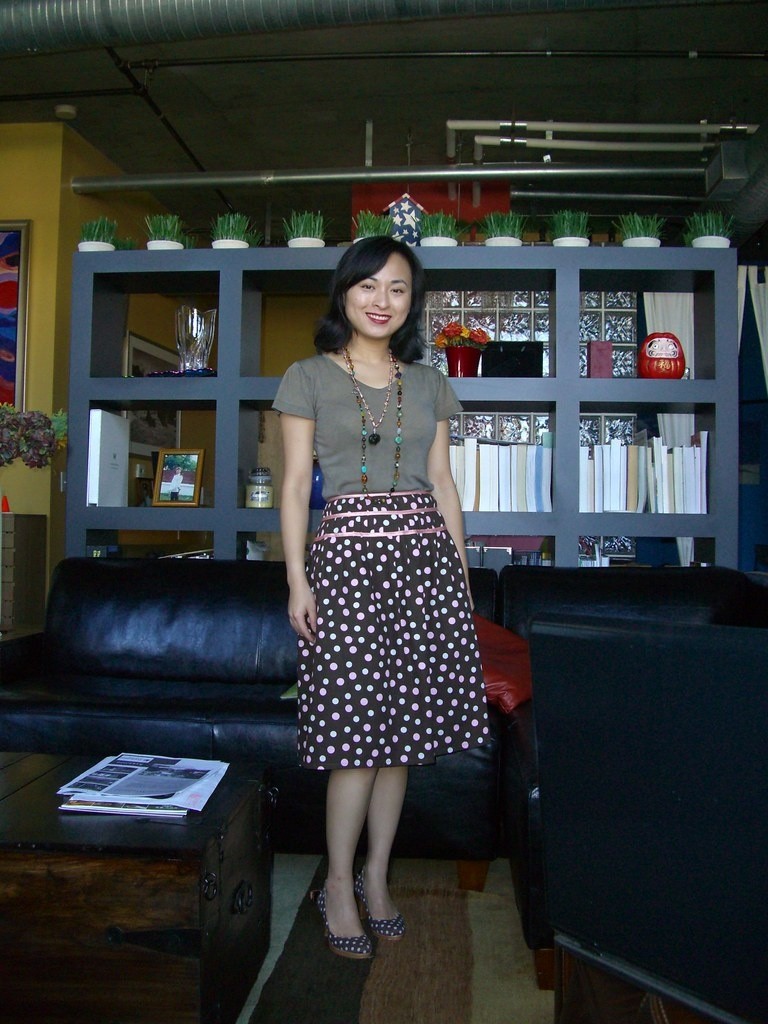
left=245, top=467, right=275, bottom=508
left=177, top=304, right=217, bottom=371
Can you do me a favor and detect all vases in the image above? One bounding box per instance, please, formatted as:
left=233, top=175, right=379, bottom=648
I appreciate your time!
left=445, top=347, right=481, bottom=378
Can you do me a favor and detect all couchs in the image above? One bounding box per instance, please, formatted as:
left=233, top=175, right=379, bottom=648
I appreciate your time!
left=0, top=558, right=768, bottom=1024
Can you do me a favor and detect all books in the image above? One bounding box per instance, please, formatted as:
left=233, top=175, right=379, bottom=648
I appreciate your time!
left=85, top=407, right=131, bottom=509
left=579, top=544, right=610, bottom=566
left=513, top=552, right=552, bottom=566
left=56, top=750, right=191, bottom=819
left=447, top=431, right=554, bottom=514
left=579, top=427, right=709, bottom=514
left=278, top=682, right=298, bottom=700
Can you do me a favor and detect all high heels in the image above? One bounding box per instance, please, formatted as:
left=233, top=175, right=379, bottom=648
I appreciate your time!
left=316, top=869, right=407, bottom=959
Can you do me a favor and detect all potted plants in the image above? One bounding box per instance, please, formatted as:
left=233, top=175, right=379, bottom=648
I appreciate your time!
left=545, top=210, right=593, bottom=246
left=611, top=212, right=667, bottom=247
left=474, top=210, right=529, bottom=247
left=77, top=216, right=118, bottom=252
left=209, top=212, right=256, bottom=249
left=282, top=209, right=335, bottom=248
left=681, top=210, right=736, bottom=248
left=131, top=214, right=186, bottom=250
left=411, top=208, right=472, bottom=247
left=352, top=209, right=407, bottom=246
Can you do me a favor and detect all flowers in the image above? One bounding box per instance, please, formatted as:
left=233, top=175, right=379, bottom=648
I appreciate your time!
left=435, top=321, right=491, bottom=352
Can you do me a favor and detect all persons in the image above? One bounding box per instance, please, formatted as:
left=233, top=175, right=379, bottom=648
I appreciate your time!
left=271, top=235, right=493, bottom=959
left=170, top=467, right=184, bottom=501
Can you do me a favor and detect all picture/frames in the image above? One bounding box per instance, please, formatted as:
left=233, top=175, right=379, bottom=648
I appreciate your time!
left=123, top=331, right=182, bottom=461
left=152, top=448, right=206, bottom=507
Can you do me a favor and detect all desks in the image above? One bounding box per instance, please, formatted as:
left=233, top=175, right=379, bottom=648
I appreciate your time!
left=0, top=749, right=278, bottom=1024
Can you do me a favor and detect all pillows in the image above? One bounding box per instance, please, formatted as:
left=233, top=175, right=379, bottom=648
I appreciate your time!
left=472, top=612, right=532, bottom=714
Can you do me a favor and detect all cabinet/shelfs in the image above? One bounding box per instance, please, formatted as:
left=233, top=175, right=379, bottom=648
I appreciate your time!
left=66, top=247, right=739, bottom=568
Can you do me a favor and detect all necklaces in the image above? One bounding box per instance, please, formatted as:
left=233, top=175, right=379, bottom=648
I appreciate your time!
left=341, top=344, right=405, bottom=504
